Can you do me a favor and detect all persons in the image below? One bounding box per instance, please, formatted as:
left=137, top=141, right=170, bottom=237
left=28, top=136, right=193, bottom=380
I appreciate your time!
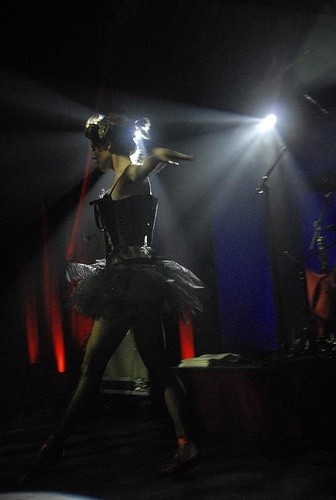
left=32, top=112, right=213, bottom=473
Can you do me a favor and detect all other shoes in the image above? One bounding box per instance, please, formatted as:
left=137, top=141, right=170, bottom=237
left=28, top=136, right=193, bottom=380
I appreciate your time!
left=17, top=437, right=66, bottom=487
left=158, top=437, right=200, bottom=476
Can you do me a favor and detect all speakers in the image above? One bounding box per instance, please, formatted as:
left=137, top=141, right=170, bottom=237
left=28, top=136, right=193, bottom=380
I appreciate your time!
left=173, top=356, right=335, bottom=452
